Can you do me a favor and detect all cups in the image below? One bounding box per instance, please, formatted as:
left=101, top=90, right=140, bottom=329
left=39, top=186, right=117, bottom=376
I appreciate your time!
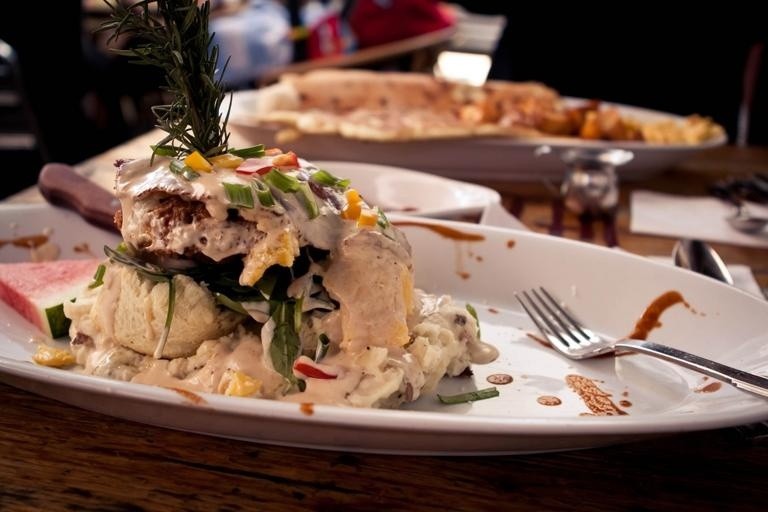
left=534, top=143, right=636, bottom=218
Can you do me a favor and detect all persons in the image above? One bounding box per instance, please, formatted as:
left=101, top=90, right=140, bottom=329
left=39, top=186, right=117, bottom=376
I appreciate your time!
left=0, top=1, right=458, bottom=201
left=455, top=1, right=767, bottom=148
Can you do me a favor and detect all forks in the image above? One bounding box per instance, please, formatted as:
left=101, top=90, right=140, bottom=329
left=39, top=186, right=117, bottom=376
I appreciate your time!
left=515, top=284, right=768, bottom=407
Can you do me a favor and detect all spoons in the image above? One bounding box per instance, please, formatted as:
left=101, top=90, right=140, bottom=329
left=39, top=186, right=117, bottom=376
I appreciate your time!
left=706, top=180, right=768, bottom=236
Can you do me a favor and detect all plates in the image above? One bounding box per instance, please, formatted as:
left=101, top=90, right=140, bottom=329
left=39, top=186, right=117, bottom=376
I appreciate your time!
left=0, top=201, right=768, bottom=458
left=292, top=158, right=503, bottom=220
left=217, top=81, right=728, bottom=184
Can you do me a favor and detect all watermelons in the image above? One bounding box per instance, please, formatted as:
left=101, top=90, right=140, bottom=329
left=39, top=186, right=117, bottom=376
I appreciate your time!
left=0, top=255, right=101, bottom=339
